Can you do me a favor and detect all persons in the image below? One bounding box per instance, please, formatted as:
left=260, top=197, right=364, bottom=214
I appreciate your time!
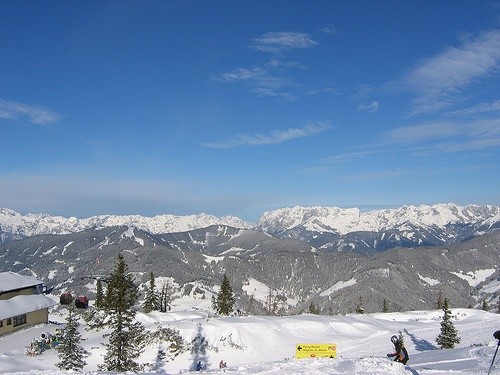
left=386, top=335, right=409, bottom=365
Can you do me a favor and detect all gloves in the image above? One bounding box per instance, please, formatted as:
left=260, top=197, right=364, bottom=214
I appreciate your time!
left=386, top=353, right=393, bottom=358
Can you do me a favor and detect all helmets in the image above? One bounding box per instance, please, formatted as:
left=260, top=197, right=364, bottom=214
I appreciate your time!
left=391, top=335, right=398, bottom=344
